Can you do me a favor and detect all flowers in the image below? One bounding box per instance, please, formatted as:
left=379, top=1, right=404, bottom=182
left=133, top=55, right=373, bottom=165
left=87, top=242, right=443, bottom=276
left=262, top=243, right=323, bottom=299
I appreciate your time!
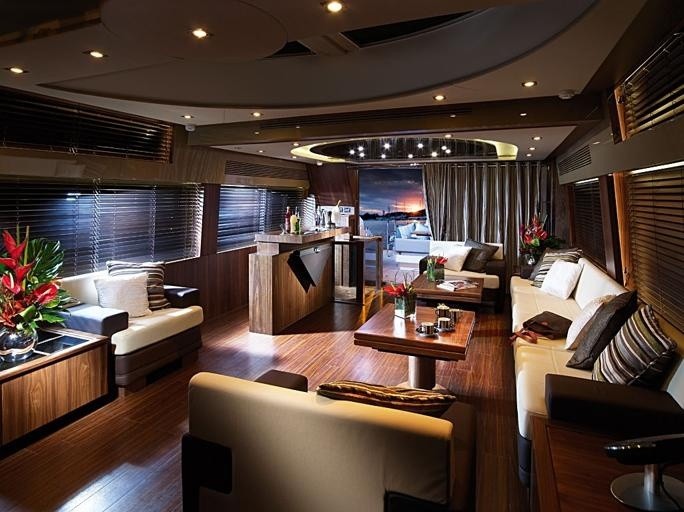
left=382, top=272, right=419, bottom=298
left=518, top=211, right=551, bottom=249
left=427, top=256, right=449, bottom=265
left=2, top=225, right=74, bottom=329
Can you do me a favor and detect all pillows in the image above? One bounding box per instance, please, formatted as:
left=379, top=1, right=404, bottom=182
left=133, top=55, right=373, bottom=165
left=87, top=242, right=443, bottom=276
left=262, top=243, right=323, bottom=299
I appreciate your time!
left=530, top=250, right=677, bottom=396
left=108, top=259, right=169, bottom=310
left=395, top=222, right=430, bottom=240
left=465, top=239, right=499, bottom=271
left=95, top=273, right=153, bottom=318
left=316, top=378, right=459, bottom=416
left=441, top=244, right=473, bottom=272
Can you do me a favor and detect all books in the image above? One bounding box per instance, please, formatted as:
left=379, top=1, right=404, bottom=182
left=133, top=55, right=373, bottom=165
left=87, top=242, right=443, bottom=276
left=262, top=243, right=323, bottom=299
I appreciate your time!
left=436, top=279, right=479, bottom=292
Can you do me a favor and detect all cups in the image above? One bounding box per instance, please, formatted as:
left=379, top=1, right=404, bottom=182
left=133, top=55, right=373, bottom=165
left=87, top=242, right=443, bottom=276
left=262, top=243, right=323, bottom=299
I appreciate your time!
left=414, top=305, right=463, bottom=337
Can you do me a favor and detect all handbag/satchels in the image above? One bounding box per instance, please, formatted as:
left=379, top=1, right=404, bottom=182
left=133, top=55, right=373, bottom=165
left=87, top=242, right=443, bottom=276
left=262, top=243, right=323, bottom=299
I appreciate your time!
left=522, top=310, right=572, bottom=340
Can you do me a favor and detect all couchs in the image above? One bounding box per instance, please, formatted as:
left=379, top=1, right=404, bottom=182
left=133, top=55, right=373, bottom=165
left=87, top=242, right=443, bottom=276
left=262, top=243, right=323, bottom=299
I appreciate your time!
left=393, top=221, right=430, bottom=255
left=176, top=368, right=479, bottom=512
left=508, top=247, right=683, bottom=486
left=429, top=238, right=508, bottom=309
left=40, top=267, right=205, bottom=394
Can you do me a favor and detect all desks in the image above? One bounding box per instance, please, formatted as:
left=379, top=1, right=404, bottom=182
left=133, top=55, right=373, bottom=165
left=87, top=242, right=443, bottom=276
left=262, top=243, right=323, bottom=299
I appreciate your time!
left=529, top=416, right=683, bottom=512
left=333, top=235, right=385, bottom=307
left=1, top=328, right=108, bottom=449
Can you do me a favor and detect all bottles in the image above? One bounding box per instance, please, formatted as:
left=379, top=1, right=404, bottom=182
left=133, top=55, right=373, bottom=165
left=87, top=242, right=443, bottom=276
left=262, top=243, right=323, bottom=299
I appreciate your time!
left=283, top=204, right=339, bottom=235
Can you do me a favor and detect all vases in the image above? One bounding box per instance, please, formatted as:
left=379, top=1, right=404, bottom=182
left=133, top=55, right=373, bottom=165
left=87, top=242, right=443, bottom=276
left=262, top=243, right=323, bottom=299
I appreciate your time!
left=1, top=317, right=37, bottom=360
left=525, top=247, right=536, bottom=264
left=395, top=292, right=416, bottom=318
left=429, top=264, right=445, bottom=282
